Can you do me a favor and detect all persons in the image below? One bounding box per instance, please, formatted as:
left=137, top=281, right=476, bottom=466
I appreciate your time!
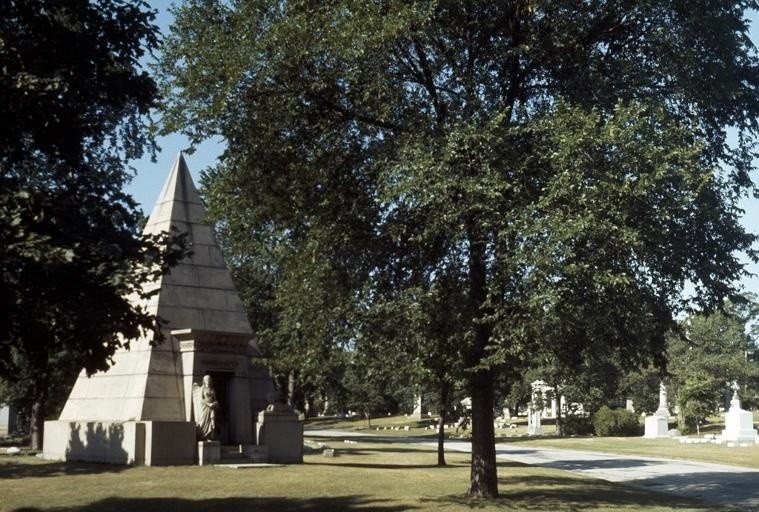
left=192, top=374, right=220, bottom=441
